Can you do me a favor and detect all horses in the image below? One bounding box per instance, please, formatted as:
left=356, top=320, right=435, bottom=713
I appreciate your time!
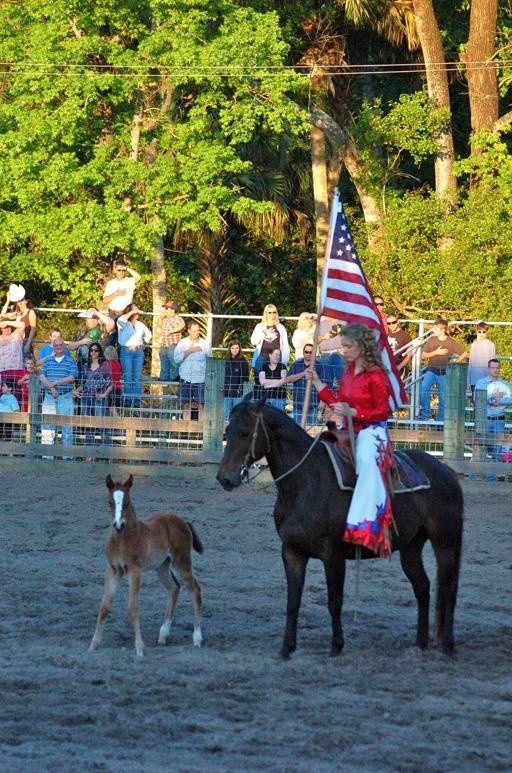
left=86, top=472, right=204, bottom=658
left=215, top=390, right=464, bottom=659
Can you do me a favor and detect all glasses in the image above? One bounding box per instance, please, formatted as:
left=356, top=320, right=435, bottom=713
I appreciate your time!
left=387, top=320, right=397, bottom=325
left=304, top=350, right=311, bottom=354
left=375, top=302, right=383, bottom=306
left=90, top=347, right=100, bottom=353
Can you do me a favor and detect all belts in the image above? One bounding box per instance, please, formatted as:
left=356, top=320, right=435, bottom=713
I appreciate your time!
left=487, top=416, right=505, bottom=420
left=124, top=345, right=142, bottom=350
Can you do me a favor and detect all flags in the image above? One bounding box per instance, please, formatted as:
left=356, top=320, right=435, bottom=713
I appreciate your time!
left=318, top=188, right=408, bottom=412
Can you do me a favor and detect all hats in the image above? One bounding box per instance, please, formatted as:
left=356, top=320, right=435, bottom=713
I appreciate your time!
left=162, top=301, right=178, bottom=310
left=9, top=284, right=25, bottom=302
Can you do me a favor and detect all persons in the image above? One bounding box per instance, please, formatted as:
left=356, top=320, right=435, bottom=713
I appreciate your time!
left=421, top=319, right=512, bottom=454
left=303, top=320, right=392, bottom=549
left=222, top=304, right=318, bottom=425
left=0, top=254, right=213, bottom=463
left=373, top=295, right=415, bottom=373
